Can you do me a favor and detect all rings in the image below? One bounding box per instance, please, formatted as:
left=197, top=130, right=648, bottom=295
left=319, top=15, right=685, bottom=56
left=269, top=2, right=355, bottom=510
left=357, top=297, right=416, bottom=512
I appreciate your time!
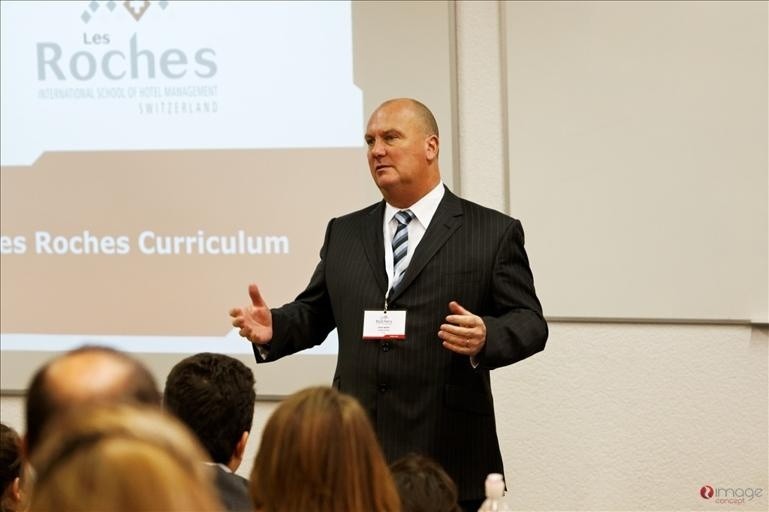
left=466, top=338, right=470, bottom=347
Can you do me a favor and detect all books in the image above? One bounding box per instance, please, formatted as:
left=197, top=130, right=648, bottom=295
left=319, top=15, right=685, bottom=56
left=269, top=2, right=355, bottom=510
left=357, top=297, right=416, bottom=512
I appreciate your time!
left=0, top=347, right=402, bottom=510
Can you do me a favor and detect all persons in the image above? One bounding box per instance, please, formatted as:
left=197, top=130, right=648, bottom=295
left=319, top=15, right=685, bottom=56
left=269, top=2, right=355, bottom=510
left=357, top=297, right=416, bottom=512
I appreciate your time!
left=229, top=98, right=547, bottom=512
left=392, top=456, right=463, bottom=512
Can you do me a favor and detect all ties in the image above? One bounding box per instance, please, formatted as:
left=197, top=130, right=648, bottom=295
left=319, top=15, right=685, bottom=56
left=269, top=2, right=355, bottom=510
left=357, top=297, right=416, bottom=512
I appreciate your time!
left=392, top=210, right=416, bottom=279
